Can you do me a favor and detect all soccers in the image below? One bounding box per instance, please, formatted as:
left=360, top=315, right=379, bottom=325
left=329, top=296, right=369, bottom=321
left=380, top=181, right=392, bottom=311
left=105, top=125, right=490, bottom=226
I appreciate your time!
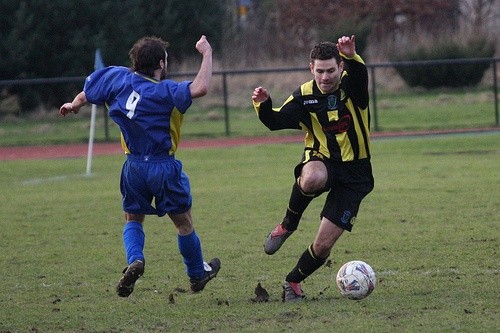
left=336, top=261, right=375, bottom=300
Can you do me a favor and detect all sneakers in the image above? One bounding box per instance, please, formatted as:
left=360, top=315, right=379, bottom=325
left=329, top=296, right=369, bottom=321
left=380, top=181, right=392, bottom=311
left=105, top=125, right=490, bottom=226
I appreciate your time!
left=190, top=258, right=221, bottom=292
left=285, top=280, right=303, bottom=301
left=264, top=221, right=297, bottom=255
left=116, top=260, right=144, bottom=297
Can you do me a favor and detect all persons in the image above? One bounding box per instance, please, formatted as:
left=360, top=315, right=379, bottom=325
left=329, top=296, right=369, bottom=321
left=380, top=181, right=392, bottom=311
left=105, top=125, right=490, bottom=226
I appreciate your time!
left=252, top=34, right=374, bottom=302
left=57, top=34, right=221, bottom=297
